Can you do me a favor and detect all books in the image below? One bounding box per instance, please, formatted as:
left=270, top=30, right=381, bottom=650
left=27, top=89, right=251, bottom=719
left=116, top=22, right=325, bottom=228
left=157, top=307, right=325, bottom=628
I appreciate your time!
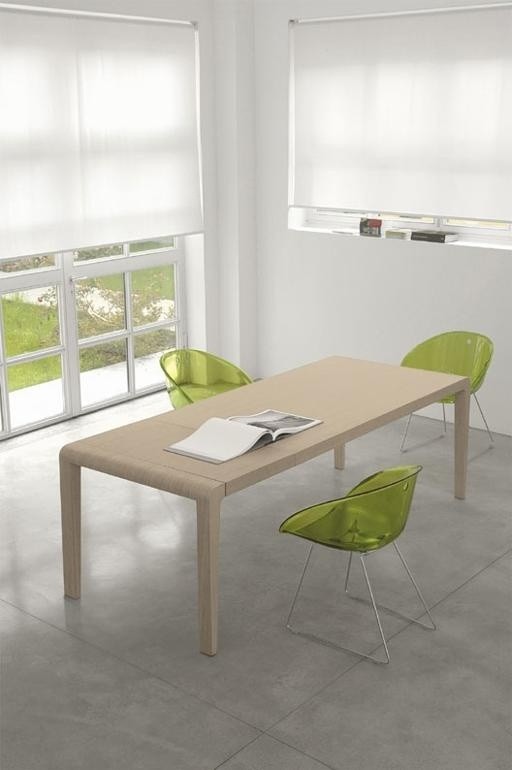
left=410, top=230, right=459, bottom=243
left=163, top=408, right=324, bottom=465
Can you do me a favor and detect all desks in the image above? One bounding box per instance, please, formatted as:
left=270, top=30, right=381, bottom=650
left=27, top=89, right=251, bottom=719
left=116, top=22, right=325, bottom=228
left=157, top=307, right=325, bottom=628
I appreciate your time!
left=61, top=355, right=472, bottom=660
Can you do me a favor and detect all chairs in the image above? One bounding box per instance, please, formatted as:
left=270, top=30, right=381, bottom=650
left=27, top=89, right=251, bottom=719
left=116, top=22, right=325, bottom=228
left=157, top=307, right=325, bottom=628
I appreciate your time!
left=159, top=347, right=263, bottom=411
left=276, top=463, right=438, bottom=663
left=399, top=328, right=497, bottom=455
